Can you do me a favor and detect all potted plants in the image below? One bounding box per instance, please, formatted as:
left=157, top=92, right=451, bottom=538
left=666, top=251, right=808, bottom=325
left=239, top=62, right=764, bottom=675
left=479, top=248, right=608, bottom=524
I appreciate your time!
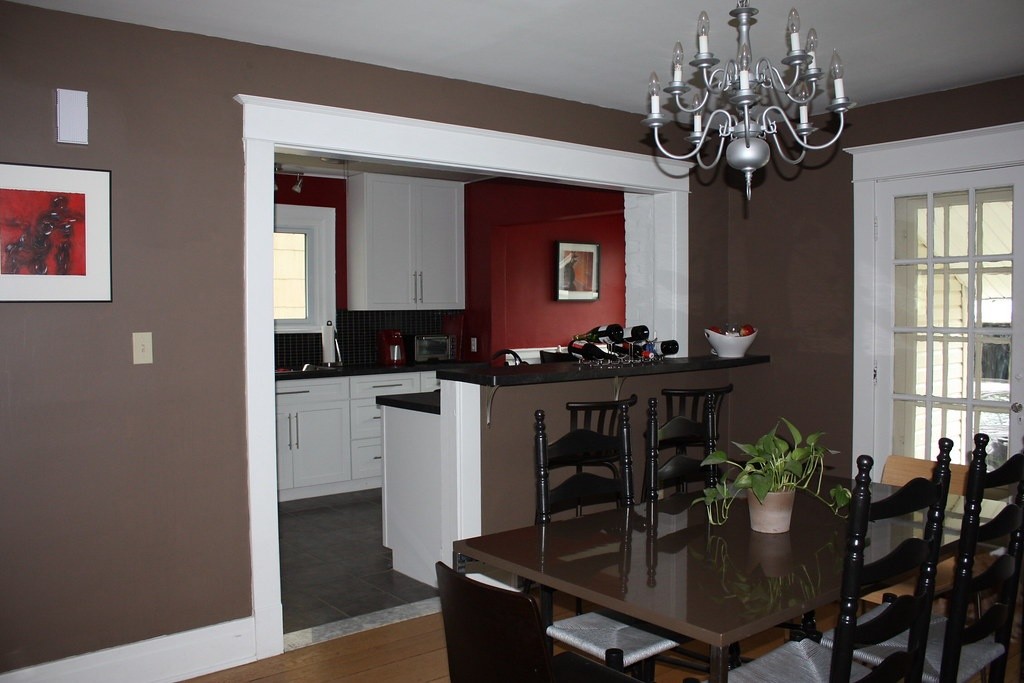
left=691, top=416, right=854, bottom=532
left=697, top=531, right=846, bottom=610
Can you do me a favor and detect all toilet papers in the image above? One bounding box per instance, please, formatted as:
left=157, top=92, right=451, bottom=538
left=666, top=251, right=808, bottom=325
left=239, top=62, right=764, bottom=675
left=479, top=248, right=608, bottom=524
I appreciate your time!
left=321, top=324, right=336, bottom=364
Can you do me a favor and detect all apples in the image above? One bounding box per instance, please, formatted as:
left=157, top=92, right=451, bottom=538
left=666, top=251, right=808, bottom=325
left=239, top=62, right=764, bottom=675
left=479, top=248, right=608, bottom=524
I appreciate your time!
left=708, top=325, right=722, bottom=334
left=740, top=324, right=754, bottom=337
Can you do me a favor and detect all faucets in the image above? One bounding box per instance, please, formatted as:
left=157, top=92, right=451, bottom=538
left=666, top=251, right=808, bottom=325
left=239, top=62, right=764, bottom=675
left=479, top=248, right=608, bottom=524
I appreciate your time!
left=490, top=350, right=522, bottom=365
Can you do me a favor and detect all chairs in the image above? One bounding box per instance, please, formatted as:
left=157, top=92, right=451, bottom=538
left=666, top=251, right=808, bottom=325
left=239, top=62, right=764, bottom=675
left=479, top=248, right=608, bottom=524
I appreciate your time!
left=534, top=405, right=681, bottom=683
left=682, top=437, right=955, bottom=683
left=435, top=561, right=645, bottom=683
left=813, top=433, right=1024, bottom=683
left=859, top=455, right=971, bottom=605
left=646, top=392, right=824, bottom=672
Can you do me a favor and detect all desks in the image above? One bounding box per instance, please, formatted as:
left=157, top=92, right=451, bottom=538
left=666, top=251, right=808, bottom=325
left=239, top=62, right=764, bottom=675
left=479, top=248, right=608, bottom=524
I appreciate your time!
left=451, top=475, right=1024, bottom=683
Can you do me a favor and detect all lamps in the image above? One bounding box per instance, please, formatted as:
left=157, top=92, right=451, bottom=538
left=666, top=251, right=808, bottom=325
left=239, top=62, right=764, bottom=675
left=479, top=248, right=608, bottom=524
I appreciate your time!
left=639, top=0, right=858, bottom=201
left=292, top=174, right=304, bottom=193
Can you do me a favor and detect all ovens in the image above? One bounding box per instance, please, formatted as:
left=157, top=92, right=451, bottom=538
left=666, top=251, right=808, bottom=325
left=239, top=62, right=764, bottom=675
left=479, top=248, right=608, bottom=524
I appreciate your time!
left=415, top=334, right=456, bottom=362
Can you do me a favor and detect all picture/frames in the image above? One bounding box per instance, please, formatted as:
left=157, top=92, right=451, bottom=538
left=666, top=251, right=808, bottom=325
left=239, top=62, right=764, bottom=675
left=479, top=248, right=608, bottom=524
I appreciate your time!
left=0, top=162, right=114, bottom=302
left=554, top=240, right=601, bottom=302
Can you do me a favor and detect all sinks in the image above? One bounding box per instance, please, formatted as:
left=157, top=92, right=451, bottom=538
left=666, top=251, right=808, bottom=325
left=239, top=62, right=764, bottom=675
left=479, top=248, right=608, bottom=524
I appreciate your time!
left=274, top=362, right=334, bottom=374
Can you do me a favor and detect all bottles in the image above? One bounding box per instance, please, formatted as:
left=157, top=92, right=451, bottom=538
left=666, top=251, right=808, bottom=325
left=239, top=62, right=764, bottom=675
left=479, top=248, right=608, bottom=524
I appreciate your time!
left=641, top=340, right=679, bottom=356
left=607, top=341, right=654, bottom=359
left=572, top=344, right=619, bottom=360
left=574, top=324, right=623, bottom=342
left=623, top=325, right=649, bottom=341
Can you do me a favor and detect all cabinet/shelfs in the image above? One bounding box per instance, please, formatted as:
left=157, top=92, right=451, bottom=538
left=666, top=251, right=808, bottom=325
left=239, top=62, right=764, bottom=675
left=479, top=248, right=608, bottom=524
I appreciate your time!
left=276, top=377, right=352, bottom=503
left=380, top=405, right=440, bottom=590
left=351, top=371, right=441, bottom=492
left=346, top=173, right=466, bottom=311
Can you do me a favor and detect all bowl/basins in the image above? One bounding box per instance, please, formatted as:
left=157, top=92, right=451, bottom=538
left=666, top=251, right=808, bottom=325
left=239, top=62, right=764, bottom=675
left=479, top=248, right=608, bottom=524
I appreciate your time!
left=703, top=328, right=758, bottom=358
